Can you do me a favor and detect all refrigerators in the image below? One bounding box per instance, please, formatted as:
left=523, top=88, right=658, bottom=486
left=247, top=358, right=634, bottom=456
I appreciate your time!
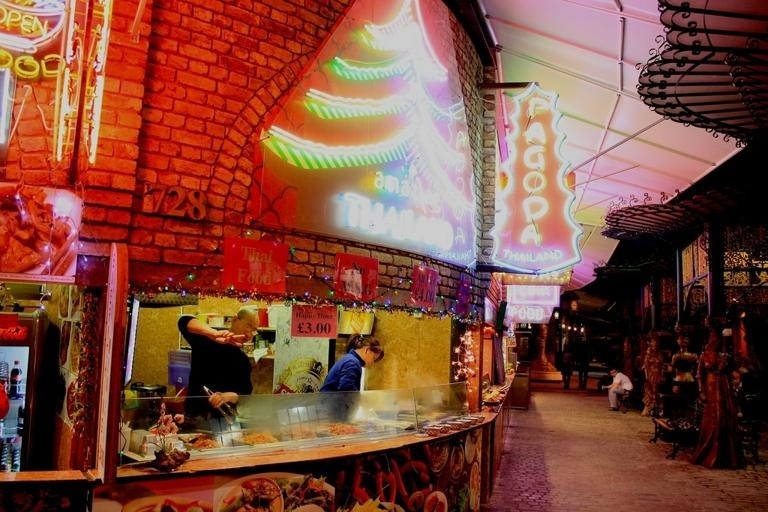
left=0, top=311, right=60, bottom=471
left=273, top=307, right=378, bottom=422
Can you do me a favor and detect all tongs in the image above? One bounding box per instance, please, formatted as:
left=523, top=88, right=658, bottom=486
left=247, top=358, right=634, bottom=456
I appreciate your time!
left=202, top=385, right=242, bottom=426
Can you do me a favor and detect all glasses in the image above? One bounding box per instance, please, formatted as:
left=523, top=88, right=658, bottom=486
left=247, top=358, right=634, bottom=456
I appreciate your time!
left=239, top=319, right=259, bottom=336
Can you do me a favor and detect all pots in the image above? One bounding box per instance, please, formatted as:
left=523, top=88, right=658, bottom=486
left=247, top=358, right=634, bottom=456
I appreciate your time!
left=130, top=381, right=167, bottom=411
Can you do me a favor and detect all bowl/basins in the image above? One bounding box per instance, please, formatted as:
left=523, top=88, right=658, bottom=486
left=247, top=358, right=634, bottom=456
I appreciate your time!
left=423, top=415, right=486, bottom=437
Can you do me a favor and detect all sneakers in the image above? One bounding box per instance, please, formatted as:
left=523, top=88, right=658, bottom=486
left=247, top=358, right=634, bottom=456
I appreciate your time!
left=608, top=406, right=618, bottom=411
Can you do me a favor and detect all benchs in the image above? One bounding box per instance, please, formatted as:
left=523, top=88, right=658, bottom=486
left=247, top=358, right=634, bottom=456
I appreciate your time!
left=652, top=417, right=702, bottom=462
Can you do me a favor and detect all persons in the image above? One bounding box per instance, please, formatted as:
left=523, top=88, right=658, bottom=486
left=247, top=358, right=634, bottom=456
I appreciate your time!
left=177, top=309, right=260, bottom=434
left=602, top=367, right=634, bottom=411
left=321, top=333, right=385, bottom=425
left=558, top=331, right=595, bottom=390
left=731, top=350, right=768, bottom=431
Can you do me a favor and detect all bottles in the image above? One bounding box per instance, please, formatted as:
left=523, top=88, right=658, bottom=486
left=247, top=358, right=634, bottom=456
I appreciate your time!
left=9, top=360, right=22, bottom=397
left=16, top=398, right=25, bottom=438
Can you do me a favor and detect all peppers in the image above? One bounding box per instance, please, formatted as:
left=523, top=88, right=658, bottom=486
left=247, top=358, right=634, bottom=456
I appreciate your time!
left=353, top=444, right=431, bottom=512
